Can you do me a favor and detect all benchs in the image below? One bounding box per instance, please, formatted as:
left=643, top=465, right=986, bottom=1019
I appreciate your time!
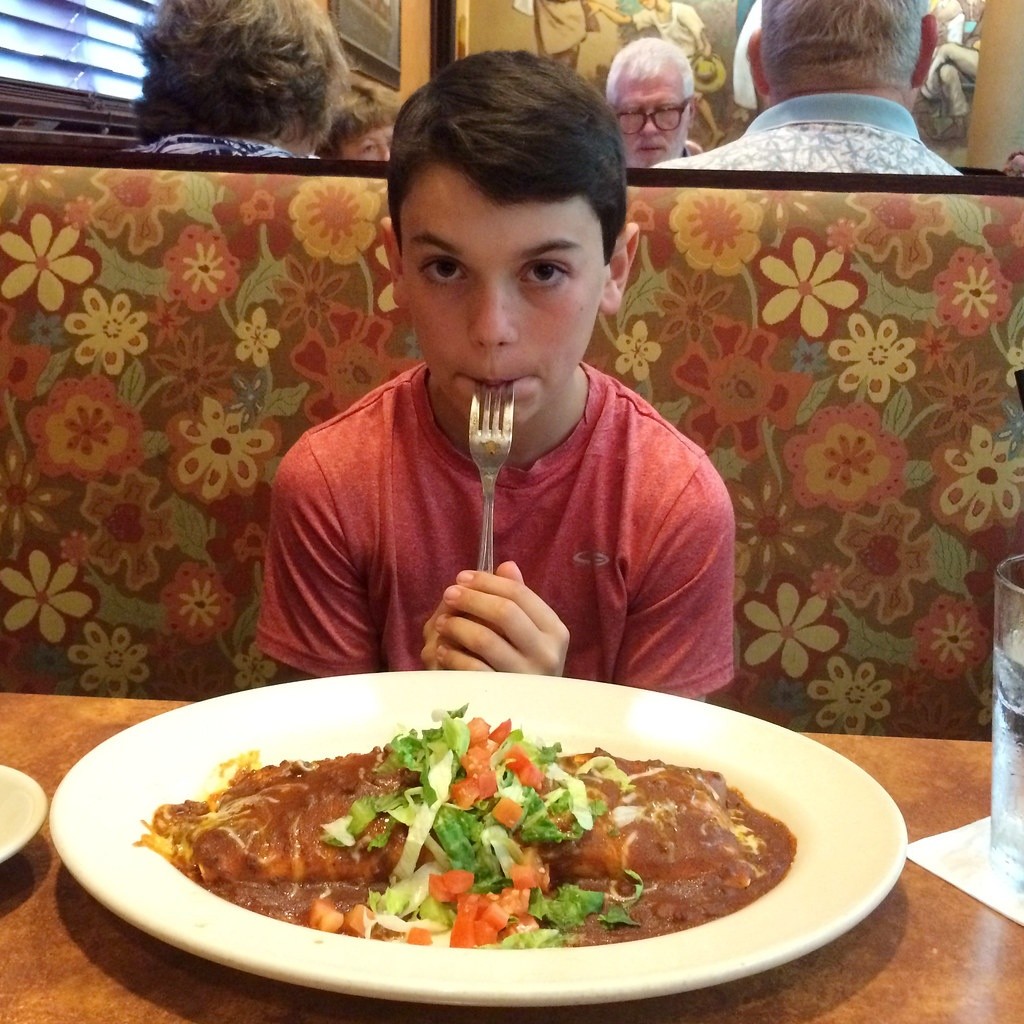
left=0, top=163, right=1023, bottom=740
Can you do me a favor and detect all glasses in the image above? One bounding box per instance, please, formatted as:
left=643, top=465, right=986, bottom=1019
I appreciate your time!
left=614, top=94, right=696, bottom=135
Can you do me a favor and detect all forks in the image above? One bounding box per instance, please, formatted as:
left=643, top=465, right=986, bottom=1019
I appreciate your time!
left=469, top=380, right=516, bottom=568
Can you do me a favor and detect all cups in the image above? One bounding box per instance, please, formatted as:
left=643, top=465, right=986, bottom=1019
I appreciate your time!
left=988, top=553, right=1024, bottom=897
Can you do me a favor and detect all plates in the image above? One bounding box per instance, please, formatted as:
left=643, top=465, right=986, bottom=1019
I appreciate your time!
left=0, top=763, right=48, bottom=864
left=48, top=670, right=911, bottom=1009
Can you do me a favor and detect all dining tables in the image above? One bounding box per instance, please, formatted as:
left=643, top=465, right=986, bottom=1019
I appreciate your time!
left=0, top=691, right=1024, bottom=1024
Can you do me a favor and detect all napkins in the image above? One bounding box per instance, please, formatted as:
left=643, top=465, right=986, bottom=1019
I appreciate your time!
left=907, top=816, right=1024, bottom=926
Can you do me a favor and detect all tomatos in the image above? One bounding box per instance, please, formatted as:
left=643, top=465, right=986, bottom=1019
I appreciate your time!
left=308, top=717, right=552, bottom=948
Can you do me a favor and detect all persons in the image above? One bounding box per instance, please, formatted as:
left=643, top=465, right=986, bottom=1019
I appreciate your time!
left=314, top=82, right=402, bottom=160
left=258, top=49, right=735, bottom=703
left=607, top=38, right=702, bottom=168
left=651, top=0, right=962, bottom=175
left=122, top=0, right=350, bottom=158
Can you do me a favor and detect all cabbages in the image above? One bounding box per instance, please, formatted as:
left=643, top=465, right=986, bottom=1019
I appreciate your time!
left=321, top=702, right=664, bottom=948
